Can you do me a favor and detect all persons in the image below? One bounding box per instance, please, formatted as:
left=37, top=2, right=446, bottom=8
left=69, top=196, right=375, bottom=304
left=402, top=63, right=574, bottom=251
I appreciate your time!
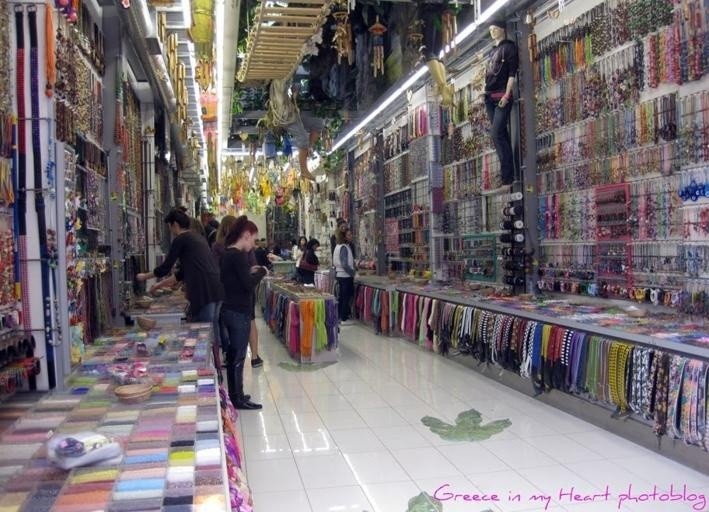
left=416, top=0, right=453, bottom=106
left=481, top=20, right=520, bottom=194
left=269, top=78, right=325, bottom=183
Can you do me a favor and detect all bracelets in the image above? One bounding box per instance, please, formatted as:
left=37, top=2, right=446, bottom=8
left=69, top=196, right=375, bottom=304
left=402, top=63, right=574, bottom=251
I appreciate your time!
left=504, top=96, right=510, bottom=102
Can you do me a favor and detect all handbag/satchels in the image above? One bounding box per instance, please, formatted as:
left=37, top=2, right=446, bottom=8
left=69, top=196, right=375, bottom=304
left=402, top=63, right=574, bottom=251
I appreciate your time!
left=298, top=258, right=318, bottom=270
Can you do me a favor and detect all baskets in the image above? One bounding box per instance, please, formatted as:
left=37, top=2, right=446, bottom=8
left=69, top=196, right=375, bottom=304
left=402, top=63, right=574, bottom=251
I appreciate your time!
left=114, top=382, right=155, bottom=405
left=626, top=309, right=646, bottom=318
left=134, top=298, right=155, bottom=308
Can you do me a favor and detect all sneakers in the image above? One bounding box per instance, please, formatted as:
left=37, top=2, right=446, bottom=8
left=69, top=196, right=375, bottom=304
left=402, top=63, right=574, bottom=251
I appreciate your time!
left=251, top=354, right=264, bottom=366
left=340, top=318, right=361, bottom=325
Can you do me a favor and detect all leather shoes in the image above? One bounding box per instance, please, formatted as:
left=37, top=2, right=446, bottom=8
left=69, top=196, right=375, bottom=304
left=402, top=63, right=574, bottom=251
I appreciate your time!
left=236, top=401, right=262, bottom=409
left=242, top=393, right=252, bottom=399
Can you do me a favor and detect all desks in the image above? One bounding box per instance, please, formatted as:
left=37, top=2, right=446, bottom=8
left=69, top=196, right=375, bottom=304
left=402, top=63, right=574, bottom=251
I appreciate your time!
left=266, top=271, right=340, bottom=365
left=313, top=266, right=709, bottom=459
left=0, top=289, right=236, bottom=512
